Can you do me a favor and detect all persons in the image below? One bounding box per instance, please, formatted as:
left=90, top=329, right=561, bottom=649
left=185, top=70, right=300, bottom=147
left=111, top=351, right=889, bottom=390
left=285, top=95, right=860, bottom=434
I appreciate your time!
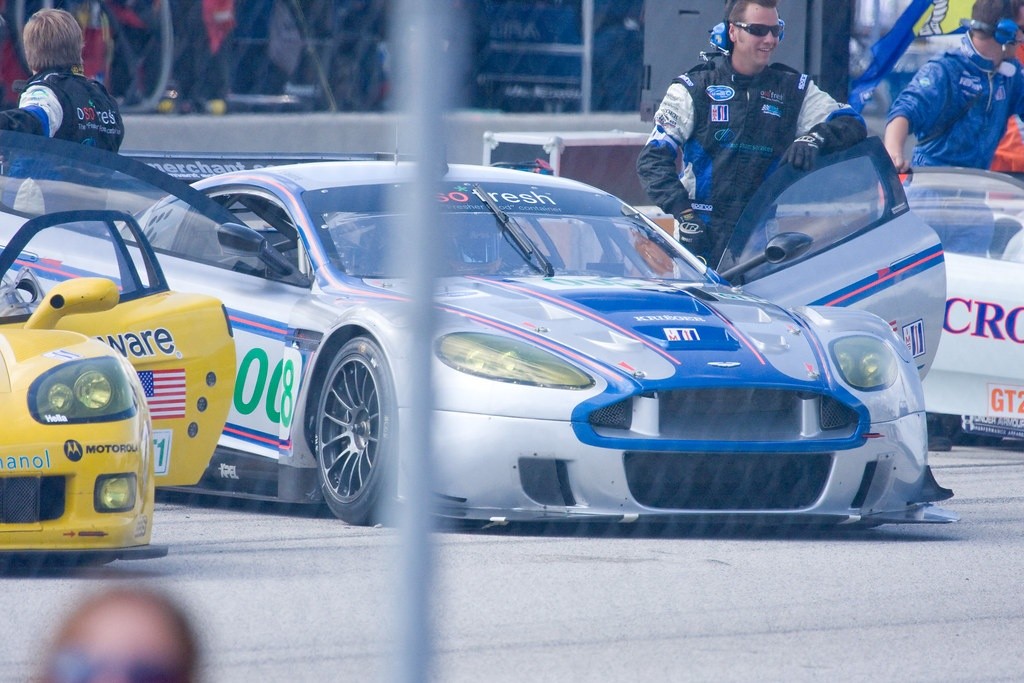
left=0, top=7, right=125, bottom=237
left=882, top=0, right=1024, bottom=174
left=635, top=0, right=868, bottom=271
left=0, top=0, right=502, bottom=125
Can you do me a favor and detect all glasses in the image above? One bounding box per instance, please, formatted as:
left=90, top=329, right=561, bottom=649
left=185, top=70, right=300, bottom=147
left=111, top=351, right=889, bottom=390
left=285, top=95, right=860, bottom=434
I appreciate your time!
left=1017, top=25, right=1023, bottom=35
left=735, top=20, right=782, bottom=38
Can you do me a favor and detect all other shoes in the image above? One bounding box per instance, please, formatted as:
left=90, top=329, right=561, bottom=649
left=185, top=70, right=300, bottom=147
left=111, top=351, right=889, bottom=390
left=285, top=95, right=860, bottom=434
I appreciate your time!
left=158, top=94, right=175, bottom=113
left=203, top=97, right=227, bottom=116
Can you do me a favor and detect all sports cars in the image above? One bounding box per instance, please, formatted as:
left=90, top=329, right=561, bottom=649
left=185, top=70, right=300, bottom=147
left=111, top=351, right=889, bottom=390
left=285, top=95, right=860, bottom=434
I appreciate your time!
left=0, top=210, right=237, bottom=570
left=875, top=165, right=1024, bottom=443
left=0, top=125, right=965, bottom=533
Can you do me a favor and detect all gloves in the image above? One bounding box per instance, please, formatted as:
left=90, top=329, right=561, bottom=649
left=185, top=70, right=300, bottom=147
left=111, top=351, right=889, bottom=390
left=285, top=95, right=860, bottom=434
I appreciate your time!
left=676, top=210, right=706, bottom=256
left=775, top=127, right=826, bottom=171
